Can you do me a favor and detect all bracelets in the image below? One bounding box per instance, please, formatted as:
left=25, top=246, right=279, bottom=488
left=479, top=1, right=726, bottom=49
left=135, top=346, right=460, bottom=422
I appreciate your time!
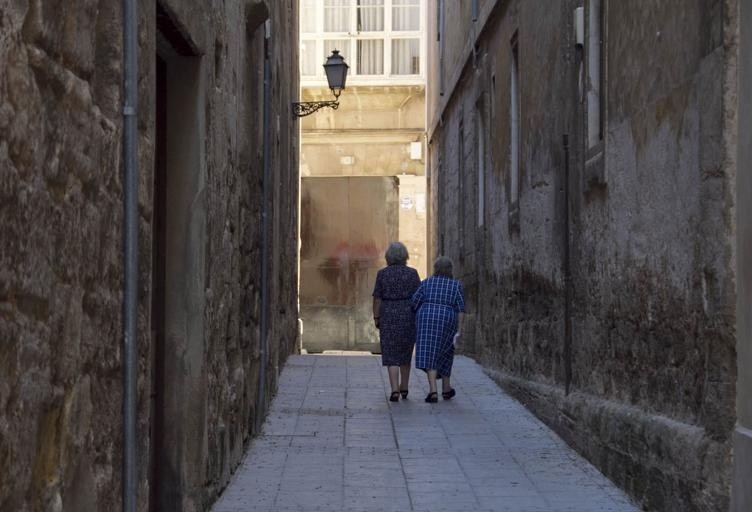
left=374, top=316, right=379, bottom=320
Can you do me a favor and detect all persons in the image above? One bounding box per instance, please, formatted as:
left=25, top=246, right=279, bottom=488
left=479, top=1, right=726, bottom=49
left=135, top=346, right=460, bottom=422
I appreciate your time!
left=372, top=241, right=421, bottom=402
left=410, top=257, right=465, bottom=402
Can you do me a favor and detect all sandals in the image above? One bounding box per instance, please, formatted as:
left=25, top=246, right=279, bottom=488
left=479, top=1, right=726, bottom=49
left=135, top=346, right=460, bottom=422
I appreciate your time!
left=442, top=387, right=457, bottom=400
left=424, top=391, right=439, bottom=403
left=389, top=388, right=409, bottom=403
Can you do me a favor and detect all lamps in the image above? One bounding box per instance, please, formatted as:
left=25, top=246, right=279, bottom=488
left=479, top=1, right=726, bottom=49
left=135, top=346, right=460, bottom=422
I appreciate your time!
left=291, top=48, right=350, bottom=118
